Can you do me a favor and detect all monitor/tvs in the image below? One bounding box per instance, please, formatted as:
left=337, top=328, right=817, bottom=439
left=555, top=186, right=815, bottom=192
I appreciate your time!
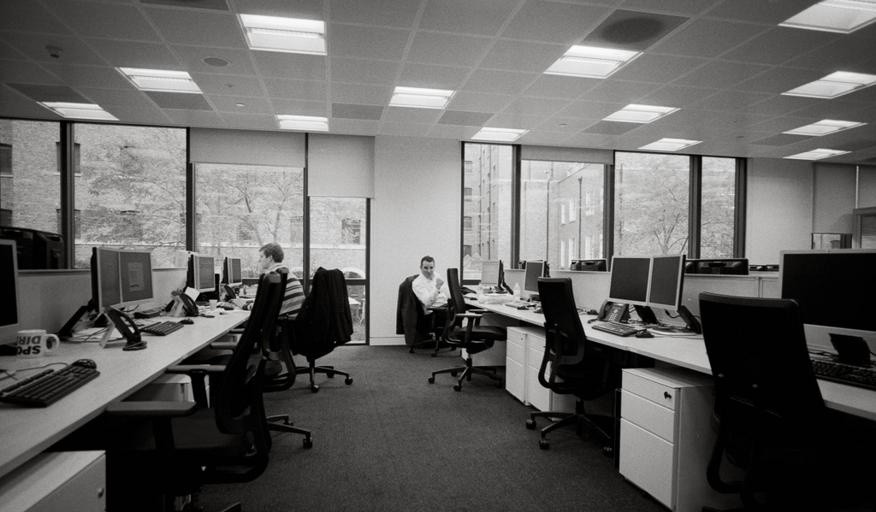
left=524, top=260, right=550, bottom=295
left=580, top=259, right=606, bottom=272
left=570, top=259, right=581, bottom=271
left=606, top=256, right=659, bottom=325
left=0, top=239, right=243, bottom=340
left=481, top=259, right=514, bottom=296
left=648, top=254, right=702, bottom=334
left=685, top=258, right=748, bottom=275
left=778, top=249, right=876, bottom=360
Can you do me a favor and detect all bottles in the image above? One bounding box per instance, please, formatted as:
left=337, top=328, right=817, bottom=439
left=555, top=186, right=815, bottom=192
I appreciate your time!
left=511, top=282, right=521, bottom=303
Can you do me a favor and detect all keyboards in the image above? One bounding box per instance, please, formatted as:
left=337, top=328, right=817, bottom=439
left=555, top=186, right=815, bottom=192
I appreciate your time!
left=592, top=320, right=647, bottom=336
left=811, top=359, right=876, bottom=391
left=1, top=366, right=101, bottom=406
left=505, top=299, right=536, bottom=307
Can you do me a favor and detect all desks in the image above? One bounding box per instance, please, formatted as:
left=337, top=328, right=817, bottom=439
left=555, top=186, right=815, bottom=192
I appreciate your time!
left=0, top=285, right=255, bottom=488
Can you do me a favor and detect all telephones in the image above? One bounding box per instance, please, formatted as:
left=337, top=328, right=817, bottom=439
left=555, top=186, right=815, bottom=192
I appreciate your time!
left=180, top=294, right=198, bottom=317
left=598, top=298, right=631, bottom=324
left=224, top=285, right=236, bottom=299
left=107, top=309, right=143, bottom=347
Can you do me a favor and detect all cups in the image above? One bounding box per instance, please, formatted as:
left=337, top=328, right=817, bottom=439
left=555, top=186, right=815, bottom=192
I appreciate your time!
left=16, top=329, right=60, bottom=359
left=209, top=299, right=217, bottom=311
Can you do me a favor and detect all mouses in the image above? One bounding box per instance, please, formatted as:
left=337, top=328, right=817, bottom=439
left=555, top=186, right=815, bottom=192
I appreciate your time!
left=635, top=329, right=652, bottom=338
left=72, top=357, right=96, bottom=369
left=470, top=296, right=477, bottom=300
left=517, top=306, right=529, bottom=310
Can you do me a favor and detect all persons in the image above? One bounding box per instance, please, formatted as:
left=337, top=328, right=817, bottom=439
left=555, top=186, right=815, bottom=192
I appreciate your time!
left=231, top=242, right=309, bottom=380
left=410, top=254, right=462, bottom=336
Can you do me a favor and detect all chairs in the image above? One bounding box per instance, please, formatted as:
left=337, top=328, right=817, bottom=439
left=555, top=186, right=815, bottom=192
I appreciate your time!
left=396, top=268, right=506, bottom=393
left=524, top=276, right=620, bottom=454
left=697, top=290, right=855, bottom=512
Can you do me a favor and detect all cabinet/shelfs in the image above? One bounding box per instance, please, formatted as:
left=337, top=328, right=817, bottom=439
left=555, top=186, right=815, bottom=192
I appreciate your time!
left=504, top=325, right=579, bottom=422
left=0, top=449, right=105, bottom=512
left=616, top=366, right=744, bottom=512
